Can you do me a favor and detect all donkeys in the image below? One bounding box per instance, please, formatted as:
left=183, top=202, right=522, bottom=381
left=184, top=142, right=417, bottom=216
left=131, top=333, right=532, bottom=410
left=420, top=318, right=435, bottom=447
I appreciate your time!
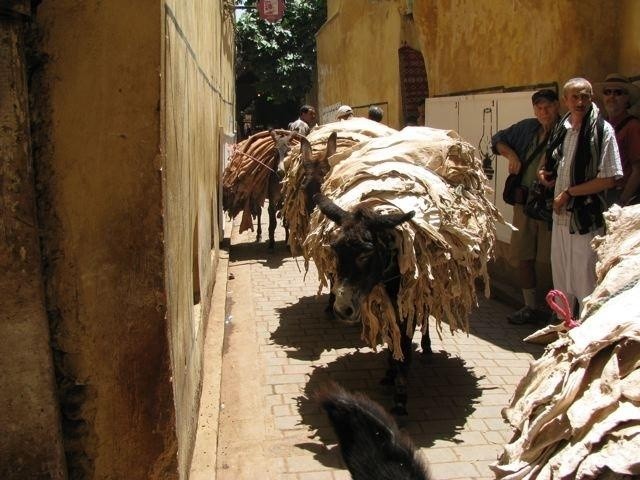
left=312, top=371, right=434, bottom=480
left=300, top=130, right=337, bottom=318
left=256, top=128, right=300, bottom=249
left=313, top=192, right=432, bottom=420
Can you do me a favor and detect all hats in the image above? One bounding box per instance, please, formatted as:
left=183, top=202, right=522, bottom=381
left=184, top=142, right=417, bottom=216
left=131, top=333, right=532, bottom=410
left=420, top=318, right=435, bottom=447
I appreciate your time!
left=336, top=105, right=353, bottom=121
left=532, top=89, right=558, bottom=105
left=594, top=74, right=640, bottom=107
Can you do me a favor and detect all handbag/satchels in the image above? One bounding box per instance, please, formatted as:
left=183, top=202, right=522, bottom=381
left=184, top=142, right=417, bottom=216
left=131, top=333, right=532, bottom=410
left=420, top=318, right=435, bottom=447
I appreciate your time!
left=503, top=173, right=521, bottom=206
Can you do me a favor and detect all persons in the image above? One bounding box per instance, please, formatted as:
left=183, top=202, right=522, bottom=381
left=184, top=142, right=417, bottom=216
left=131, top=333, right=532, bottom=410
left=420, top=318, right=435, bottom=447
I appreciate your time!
left=490, top=89, right=565, bottom=325
left=537, top=77, right=624, bottom=319
left=595, top=72, right=640, bottom=235
left=289, top=104, right=384, bottom=137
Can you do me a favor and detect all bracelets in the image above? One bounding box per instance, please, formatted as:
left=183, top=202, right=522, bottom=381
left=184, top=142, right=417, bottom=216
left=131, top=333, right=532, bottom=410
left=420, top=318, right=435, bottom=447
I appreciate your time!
left=565, top=190, right=573, bottom=198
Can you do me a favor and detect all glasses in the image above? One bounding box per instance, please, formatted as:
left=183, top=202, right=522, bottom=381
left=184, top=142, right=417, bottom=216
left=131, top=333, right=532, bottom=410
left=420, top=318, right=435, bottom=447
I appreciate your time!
left=603, top=88, right=627, bottom=97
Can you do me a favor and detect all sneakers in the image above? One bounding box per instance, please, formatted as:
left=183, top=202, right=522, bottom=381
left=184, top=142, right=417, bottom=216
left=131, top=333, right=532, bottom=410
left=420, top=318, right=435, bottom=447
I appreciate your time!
left=506, top=304, right=539, bottom=325
